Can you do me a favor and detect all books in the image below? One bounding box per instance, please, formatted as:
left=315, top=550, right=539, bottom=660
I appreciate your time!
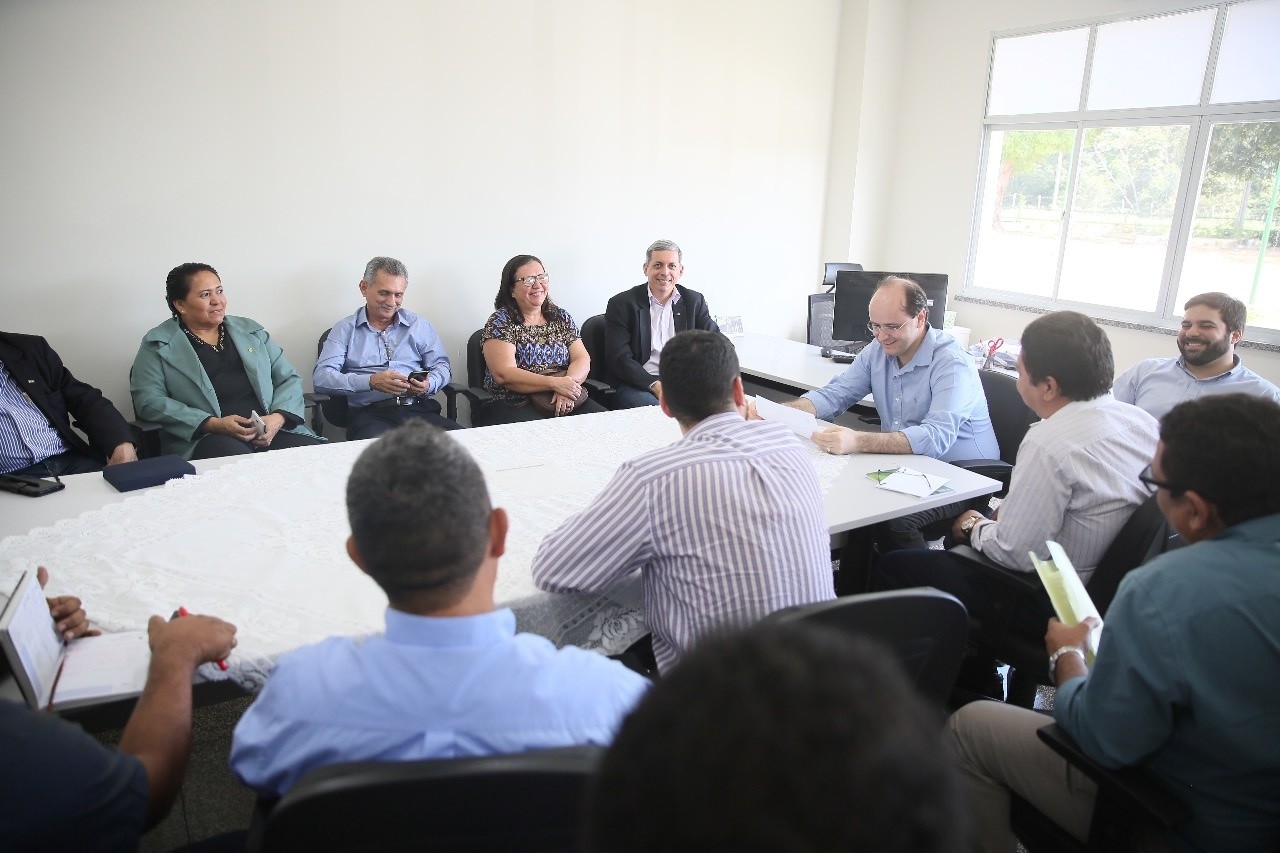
left=0, top=566, right=154, bottom=716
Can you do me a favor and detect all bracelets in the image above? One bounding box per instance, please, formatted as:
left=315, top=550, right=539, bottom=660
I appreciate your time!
left=1047, top=645, right=1088, bottom=687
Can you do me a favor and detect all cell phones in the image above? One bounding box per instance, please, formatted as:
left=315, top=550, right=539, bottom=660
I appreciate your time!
left=250, top=410, right=266, bottom=438
left=0, top=474, right=65, bottom=497
left=406, top=371, right=429, bottom=386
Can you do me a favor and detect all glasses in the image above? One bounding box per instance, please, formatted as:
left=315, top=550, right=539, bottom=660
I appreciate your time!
left=512, top=274, right=549, bottom=286
left=1139, top=462, right=1186, bottom=495
left=867, top=316, right=913, bottom=334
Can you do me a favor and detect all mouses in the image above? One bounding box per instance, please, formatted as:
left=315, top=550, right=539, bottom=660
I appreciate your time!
left=821, top=349, right=832, bottom=358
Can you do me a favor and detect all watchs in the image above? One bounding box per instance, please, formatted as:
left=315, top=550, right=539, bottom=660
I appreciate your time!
left=959, top=513, right=983, bottom=537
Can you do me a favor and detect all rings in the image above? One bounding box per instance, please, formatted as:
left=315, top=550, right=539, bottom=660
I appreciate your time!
left=826, top=446, right=831, bottom=454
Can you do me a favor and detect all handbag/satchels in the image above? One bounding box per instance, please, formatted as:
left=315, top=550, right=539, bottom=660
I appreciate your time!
left=531, top=368, right=589, bottom=417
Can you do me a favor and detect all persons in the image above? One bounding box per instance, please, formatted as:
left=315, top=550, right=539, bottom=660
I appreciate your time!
left=312, top=255, right=466, bottom=440
left=747, top=274, right=1001, bottom=563
left=233, top=420, right=651, bottom=802
left=870, top=309, right=1170, bottom=627
left=481, top=254, right=608, bottom=423
left=532, top=329, right=839, bottom=677
left=937, top=392, right=1279, bottom=848
left=0, top=333, right=139, bottom=481
left=609, top=240, right=728, bottom=408
left=132, top=262, right=325, bottom=460
left=1113, top=291, right=1279, bottom=426
left=0, top=564, right=239, bottom=852
left=602, top=617, right=976, bottom=852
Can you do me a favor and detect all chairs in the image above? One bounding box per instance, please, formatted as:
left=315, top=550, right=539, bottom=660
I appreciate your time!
left=752, top=585, right=972, bottom=720
left=1008, top=721, right=1194, bottom=853
left=246, top=744, right=610, bottom=853
left=72, top=312, right=615, bottom=459
left=947, top=460, right=1189, bottom=710
left=807, top=262, right=864, bottom=354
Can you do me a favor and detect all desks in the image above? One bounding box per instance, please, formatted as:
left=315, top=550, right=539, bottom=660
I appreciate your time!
left=977, top=363, right=1042, bottom=499
left=730, top=332, right=880, bottom=418
left=1, top=391, right=1003, bottom=728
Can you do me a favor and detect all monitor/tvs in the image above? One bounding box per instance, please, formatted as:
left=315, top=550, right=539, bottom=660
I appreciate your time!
left=831, top=271, right=948, bottom=342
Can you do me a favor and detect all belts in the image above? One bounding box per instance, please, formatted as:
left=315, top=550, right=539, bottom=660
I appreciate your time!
left=370, top=396, right=422, bottom=406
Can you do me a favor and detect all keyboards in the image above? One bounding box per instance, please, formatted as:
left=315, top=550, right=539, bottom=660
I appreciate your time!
left=831, top=354, right=856, bottom=364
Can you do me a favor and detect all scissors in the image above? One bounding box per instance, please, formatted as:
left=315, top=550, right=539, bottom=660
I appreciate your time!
left=988, top=337, right=1004, bottom=355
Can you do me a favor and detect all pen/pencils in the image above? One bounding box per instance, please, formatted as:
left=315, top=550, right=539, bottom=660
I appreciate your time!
left=177, top=606, right=227, bottom=671
